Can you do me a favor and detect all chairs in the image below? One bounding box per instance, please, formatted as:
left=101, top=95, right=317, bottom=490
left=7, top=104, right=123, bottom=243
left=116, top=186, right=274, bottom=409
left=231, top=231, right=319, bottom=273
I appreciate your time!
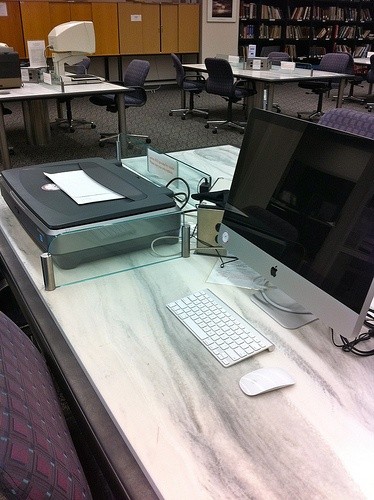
left=49, top=53, right=374, bottom=149
left=0, top=311, right=91, bottom=500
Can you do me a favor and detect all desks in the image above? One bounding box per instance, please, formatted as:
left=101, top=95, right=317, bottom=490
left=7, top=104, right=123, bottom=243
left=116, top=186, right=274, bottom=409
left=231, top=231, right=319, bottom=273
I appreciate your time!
left=0, top=69, right=129, bottom=170
left=0, top=144, right=374, bottom=500
left=181, top=62, right=355, bottom=126
left=353, top=58, right=372, bottom=101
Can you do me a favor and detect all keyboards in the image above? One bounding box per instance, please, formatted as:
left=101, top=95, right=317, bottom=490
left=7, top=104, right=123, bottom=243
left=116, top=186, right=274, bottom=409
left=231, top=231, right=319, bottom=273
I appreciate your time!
left=167, top=288, right=276, bottom=368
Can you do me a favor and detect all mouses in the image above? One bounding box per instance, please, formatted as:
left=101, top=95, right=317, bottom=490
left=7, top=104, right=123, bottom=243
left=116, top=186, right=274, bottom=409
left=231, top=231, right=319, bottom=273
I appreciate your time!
left=238, top=368, right=295, bottom=395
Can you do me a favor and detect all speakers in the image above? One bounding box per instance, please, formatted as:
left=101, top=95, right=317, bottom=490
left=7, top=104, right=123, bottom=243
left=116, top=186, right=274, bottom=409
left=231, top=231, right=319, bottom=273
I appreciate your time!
left=195, top=207, right=228, bottom=255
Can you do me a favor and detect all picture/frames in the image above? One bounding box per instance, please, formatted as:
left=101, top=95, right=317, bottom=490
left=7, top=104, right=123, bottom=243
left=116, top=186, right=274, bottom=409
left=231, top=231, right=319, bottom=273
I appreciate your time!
left=207, top=0, right=237, bottom=23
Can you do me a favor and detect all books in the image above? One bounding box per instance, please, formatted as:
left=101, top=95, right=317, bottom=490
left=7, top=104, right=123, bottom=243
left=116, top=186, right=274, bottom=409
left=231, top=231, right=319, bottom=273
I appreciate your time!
left=240, top=3, right=374, bottom=72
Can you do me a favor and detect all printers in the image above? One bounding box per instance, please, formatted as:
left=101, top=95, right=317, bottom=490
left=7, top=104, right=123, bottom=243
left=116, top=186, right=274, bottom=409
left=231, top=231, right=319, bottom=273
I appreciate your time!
left=0, top=156, right=181, bottom=269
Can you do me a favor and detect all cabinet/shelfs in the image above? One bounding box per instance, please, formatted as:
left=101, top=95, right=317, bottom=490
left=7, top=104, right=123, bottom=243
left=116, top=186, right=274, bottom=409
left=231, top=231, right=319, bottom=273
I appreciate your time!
left=238, top=0, right=374, bottom=59
left=0, top=0, right=200, bottom=60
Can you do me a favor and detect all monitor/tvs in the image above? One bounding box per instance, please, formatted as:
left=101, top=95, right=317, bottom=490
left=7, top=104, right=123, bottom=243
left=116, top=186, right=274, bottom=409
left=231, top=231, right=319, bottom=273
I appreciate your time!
left=218, top=107, right=374, bottom=338
left=48, top=21, right=95, bottom=77
